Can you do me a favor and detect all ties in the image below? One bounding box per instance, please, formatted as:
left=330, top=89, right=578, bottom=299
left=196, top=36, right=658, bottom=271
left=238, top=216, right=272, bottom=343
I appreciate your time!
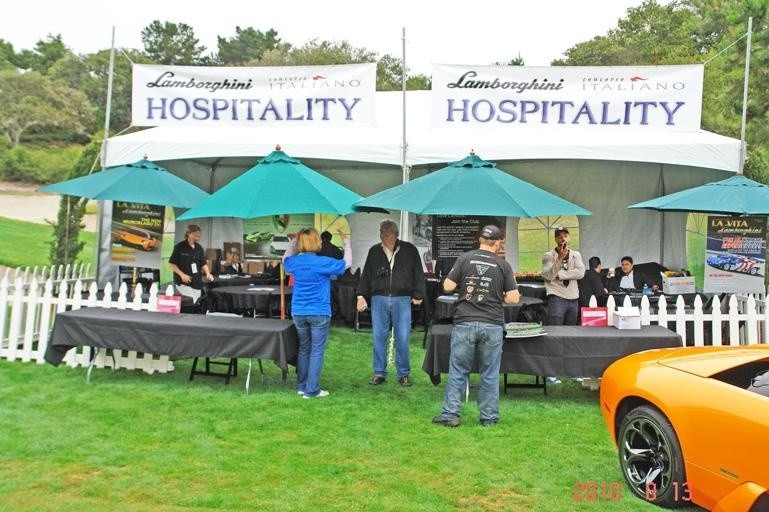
left=563, top=259, right=570, bottom=288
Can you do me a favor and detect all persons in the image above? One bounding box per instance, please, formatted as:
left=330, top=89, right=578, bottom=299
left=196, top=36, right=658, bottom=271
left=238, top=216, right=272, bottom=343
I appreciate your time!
left=279, top=223, right=351, bottom=400
left=601, top=255, right=650, bottom=293
left=431, top=226, right=520, bottom=428
left=539, top=227, right=586, bottom=387
left=307, top=227, right=345, bottom=262
left=167, top=225, right=214, bottom=297
left=221, top=246, right=244, bottom=280
left=578, top=256, right=609, bottom=309
left=356, top=221, right=425, bottom=388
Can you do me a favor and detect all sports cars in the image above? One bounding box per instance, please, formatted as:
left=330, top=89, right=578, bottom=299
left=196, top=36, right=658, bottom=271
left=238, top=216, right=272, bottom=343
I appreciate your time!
left=116, top=225, right=158, bottom=252
left=706, top=253, right=763, bottom=276
left=244, top=227, right=301, bottom=255
left=596, top=343, right=768, bottom=510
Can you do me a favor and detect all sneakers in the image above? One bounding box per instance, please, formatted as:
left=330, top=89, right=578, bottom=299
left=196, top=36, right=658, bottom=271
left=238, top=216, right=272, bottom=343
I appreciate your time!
left=432, top=414, right=460, bottom=427
left=369, top=374, right=385, bottom=385
left=399, top=374, right=411, bottom=387
left=297, top=388, right=329, bottom=399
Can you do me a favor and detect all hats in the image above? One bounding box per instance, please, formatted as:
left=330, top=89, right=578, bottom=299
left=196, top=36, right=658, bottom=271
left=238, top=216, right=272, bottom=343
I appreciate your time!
left=480, top=224, right=502, bottom=240
left=555, top=226, right=569, bottom=235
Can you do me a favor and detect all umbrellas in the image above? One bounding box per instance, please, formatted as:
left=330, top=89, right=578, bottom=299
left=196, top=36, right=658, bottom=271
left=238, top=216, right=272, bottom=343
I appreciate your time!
left=623, top=173, right=768, bottom=219
left=173, top=143, right=394, bottom=320
left=354, top=149, right=596, bottom=224
left=33, top=152, right=211, bottom=300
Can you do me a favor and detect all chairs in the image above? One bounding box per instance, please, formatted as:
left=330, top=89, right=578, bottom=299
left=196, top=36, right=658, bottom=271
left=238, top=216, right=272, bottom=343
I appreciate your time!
left=677, top=293, right=708, bottom=305
left=504, top=320, right=547, bottom=395
left=352, top=288, right=372, bottom=332
left=704, top=293, right=730, bottom=345
left=189, top=309, right=244, bottom=385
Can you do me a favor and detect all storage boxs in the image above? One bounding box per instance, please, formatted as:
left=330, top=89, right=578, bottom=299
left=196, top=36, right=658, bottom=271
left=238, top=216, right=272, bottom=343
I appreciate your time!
left=660, top=272, right=696, bottom=294
left=613, top=311, right=641, bottom=329
left=580, top=307, right=608, bottom=327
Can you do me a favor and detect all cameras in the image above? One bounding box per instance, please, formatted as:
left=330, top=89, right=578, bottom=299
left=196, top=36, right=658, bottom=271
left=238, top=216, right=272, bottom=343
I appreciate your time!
left=376, top=265, right=390, bottom=276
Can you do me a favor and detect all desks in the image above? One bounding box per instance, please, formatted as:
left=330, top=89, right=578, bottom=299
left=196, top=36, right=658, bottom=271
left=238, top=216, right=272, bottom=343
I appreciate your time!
left=66, top=291, right=193, bottom=307
left=423, top=278, right=546, bottom=348
left=211, top=285, right=294, bottom=318
left=338, top=286, right=373, bottom=328
left=422, top=325, right=683, bottom=389
left=650, top=309, right=712, bottom=345
left=213, top=273, right=288, bottom=288
left=44, top=307, right=299, bottom=395
left=435, top=293, right=548, bottom=324
left=605, top=292, right=676, bottom=306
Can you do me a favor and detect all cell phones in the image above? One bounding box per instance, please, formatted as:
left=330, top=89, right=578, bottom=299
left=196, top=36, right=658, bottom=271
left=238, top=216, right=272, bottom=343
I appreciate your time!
left=561, top=242, right=566, bottom=248
